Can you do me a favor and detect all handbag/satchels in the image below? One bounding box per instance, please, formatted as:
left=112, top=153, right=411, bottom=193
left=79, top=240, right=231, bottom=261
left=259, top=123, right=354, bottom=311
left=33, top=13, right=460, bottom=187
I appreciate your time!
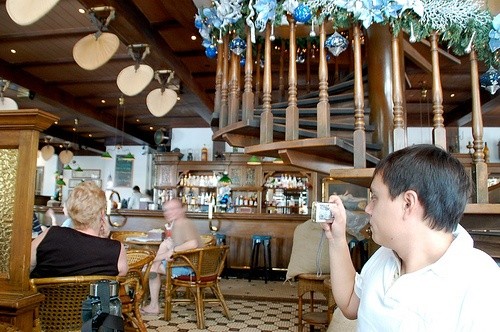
left=281, top=218, right=331, bottom=284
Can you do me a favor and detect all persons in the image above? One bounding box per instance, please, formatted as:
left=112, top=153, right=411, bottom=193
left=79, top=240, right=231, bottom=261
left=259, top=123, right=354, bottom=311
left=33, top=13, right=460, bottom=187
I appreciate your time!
left=32, top=210, right=43, bottom=239
left=30, top=181, right=128, bottom=279
left=319, top=143, right=500, bottom=332
left=138, top=198, right=200, bottom=315
left=128, top=186, right=145, bottom=209
left=41, top=199, right=75, bottom=231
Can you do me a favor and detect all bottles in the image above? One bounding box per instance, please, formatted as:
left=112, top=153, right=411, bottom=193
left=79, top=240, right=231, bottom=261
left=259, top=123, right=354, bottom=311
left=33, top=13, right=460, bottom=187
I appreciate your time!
left=217, top=194, right=234, bottom=214
left=157, top=188, right=177, bottom=206
left=264, top=173, right=309, bottom=215
left=236, top=207, right=256, bottom=214
left=187, top=148, right=194, bottom=161
left=179, top=173, right=221, bottom=188
left=201, top=144, right=208, bottom=161
left=217, top=171, right=232, bottom=194
left=178, top=188, right=217, bottom=206
left=235, top=194, right=258, bottom=207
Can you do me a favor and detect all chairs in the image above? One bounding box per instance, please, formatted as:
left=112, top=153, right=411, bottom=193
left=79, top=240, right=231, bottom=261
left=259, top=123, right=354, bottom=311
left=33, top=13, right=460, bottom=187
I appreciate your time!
left=28, top=231, right=231, bottom=332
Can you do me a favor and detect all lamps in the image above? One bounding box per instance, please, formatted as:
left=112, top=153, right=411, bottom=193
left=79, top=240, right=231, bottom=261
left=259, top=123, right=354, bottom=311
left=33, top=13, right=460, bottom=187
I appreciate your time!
left=41, top=136, right=54, bottom=161
left=246, top=154, right=262, bottom=165
left=116, top=44, right=155, bottom=97
left=217, top=173, right=232, bottom=186
left=0, top=79, right=19, bottom=110
left=72, top=6, right=120, bottom=71
left=101, top=93, right=136, bottom=160
left=146, top=70, right=180, bottom=117
left=55, top=160, right=83, bottom=187
left=59, top=142, right=74, bottom=163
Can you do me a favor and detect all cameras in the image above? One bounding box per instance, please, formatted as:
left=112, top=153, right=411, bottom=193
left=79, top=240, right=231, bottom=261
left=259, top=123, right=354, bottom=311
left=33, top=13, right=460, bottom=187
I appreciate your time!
left=311, top=201, right=336, bottom=223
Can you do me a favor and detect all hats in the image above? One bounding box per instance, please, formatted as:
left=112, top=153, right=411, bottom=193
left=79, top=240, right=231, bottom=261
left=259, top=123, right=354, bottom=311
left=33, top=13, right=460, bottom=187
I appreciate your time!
left=134, top=186, right=139, bottom=191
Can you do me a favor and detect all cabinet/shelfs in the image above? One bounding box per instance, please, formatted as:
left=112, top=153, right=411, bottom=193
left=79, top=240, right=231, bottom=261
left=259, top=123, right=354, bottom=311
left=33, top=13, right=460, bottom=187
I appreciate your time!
left=154, top=152, right=312, bottom=213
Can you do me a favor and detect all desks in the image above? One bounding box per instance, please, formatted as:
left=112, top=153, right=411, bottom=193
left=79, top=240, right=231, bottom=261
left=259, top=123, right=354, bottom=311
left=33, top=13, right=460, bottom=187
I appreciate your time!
left=123, top=238, right=161, bottom=252
left=296, top=274, right=335, bottom=332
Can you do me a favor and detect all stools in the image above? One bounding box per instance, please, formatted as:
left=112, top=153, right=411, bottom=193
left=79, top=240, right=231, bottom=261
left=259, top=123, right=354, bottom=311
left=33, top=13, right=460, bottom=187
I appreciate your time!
left=215, top=234, right=229, bottom=280
left=248, top=234, right=273, bottom=284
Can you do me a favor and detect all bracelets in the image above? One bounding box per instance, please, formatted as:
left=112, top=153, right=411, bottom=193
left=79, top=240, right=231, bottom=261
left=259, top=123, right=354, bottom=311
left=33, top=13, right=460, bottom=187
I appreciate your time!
left=173, top=247, right=177, bottom=253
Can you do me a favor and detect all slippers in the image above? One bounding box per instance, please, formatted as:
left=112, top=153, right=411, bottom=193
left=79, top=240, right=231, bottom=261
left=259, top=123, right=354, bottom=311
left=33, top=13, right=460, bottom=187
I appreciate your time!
left=142, top=258, right=166, bottom=272
left=139, top=306, right=160, bottom=316
